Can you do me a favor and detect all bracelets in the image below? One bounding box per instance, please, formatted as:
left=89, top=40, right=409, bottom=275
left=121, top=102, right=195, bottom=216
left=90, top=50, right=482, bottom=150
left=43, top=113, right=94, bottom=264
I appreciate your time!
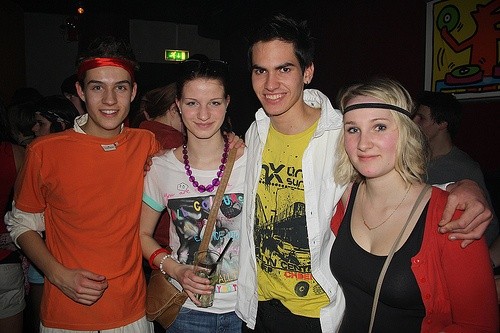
left=160, top=255, right=180, bottom=274
left=149, top=248, right=171, bottom=270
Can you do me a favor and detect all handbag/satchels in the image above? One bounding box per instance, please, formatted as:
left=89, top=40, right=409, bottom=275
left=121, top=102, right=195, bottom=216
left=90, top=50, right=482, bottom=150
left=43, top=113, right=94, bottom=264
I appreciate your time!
left=144, top=268, right=186, bottom=331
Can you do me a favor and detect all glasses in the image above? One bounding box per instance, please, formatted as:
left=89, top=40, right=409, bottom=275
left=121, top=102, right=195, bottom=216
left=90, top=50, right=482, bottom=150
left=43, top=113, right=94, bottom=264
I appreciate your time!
left=180, top=59, right=230, bottom=75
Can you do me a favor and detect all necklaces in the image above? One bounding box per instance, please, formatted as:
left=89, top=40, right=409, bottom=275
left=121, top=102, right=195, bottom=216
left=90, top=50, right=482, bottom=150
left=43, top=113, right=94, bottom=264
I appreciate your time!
left=183, top=133, right=230, bottom=193
left=361, top=177, right=412, bottom=230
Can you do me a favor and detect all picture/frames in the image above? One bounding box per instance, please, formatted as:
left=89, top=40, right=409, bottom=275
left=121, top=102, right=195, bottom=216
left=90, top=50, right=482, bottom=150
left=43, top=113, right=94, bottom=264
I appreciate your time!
left=422, top=0, right=500, bottom=102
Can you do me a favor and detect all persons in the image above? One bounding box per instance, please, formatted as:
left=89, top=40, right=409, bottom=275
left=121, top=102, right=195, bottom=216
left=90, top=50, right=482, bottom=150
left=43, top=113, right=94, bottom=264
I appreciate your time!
left=139, top=61, right=247, bottom=333
left=412, top=91, right=499, bottom=248
left=327, top=78, right=499, bottom=333
left=123, top=48, right=210, bottom=286
left=0, top=74, right=87, bottom=333
left=142, top=15, right=494, bottom=333
left=4, top=37, right=245, bottom=333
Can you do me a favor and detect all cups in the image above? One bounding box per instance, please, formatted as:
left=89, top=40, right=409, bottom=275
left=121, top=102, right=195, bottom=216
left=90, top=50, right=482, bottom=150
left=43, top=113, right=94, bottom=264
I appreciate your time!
left=193, top=249, right=223, bottom=308
